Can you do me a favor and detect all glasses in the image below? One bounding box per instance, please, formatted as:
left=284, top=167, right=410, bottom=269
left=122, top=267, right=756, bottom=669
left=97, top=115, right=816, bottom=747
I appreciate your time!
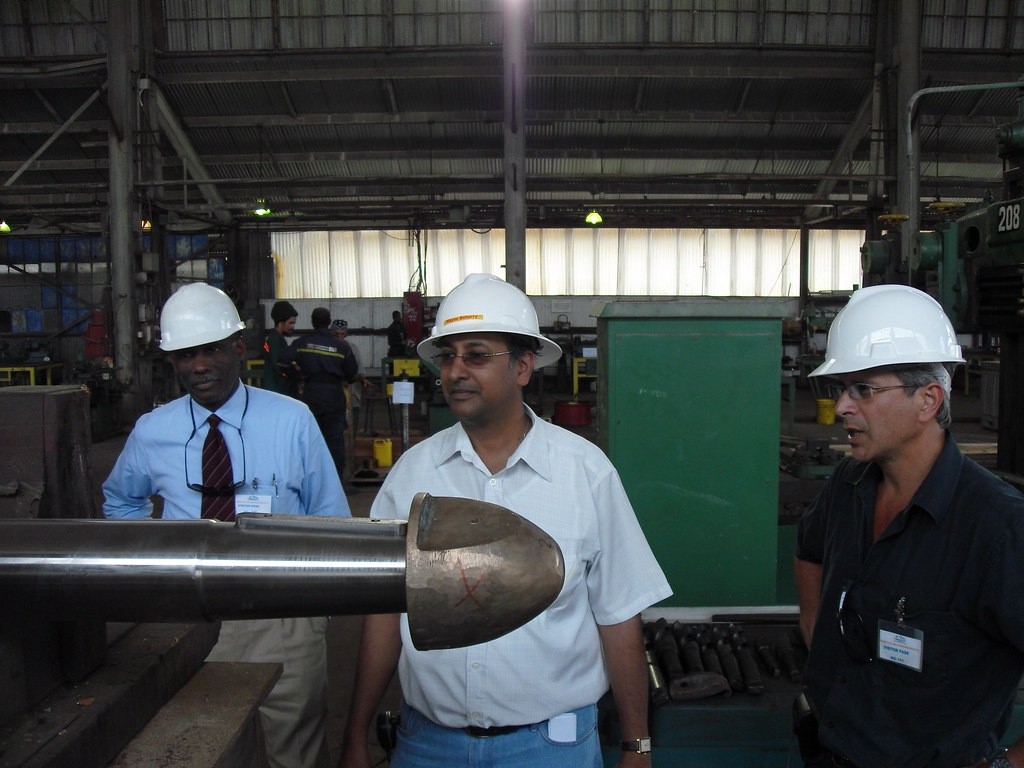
left=184, top=429, right=247, bottom=492
left=430, top=350, right=513, bottom=370
left=834, top=382, right=915, bottom=399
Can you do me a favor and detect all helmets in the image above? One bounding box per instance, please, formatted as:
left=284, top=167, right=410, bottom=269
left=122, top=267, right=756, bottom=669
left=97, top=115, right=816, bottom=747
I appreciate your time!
left=416, top=273, right=563, bottom=370
left=159, top=282, right=246, bottom=351
left=808, top=285, right=967, bottom=376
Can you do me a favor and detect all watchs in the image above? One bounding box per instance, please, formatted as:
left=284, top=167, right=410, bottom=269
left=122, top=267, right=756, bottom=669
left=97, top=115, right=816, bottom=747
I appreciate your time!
left=621, top=737, right=654, bottom=756
left=985, top=748, right=1014, bottom=768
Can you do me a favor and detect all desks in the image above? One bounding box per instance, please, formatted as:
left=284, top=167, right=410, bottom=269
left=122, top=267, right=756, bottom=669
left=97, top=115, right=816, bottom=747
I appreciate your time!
left=0, top=363, right=64, bottom=387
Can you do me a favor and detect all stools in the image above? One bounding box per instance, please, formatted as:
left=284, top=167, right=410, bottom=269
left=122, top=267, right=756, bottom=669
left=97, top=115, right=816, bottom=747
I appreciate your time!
left=365, top=395, right=397, bottom=434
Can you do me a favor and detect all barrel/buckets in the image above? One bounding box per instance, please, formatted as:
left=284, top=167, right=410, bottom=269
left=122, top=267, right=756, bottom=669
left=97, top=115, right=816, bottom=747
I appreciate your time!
left=817, top=399, right=836, bottom=425
left=373, top=439, right=392, bottom=467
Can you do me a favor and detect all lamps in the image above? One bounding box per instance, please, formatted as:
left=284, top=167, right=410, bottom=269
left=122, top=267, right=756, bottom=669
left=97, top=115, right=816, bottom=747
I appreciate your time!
left=253, top=125, right=270, bottom=215
left=0, top=221, right=11, bottom=232
left=585, top=190, right=603, bottom=224
left=933, top=126, right=942, bottom=203
left=142, top=220, right=151, bottom=231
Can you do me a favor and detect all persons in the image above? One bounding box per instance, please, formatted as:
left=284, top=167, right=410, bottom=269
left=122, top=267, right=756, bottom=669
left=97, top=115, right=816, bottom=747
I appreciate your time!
left=260, top=301, right=377, bottom=493
left=792, top=284, right=1024, bottom=768
left=387, top=311, right=408, bottom=383
left=339, top=270, right=674, bottom=768
left=101, top=282, right=352, bottom=768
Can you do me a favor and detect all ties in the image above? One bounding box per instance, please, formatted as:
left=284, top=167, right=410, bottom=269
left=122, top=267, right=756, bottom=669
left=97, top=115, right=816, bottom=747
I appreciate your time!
left=202, top=412, right=236, bottom=522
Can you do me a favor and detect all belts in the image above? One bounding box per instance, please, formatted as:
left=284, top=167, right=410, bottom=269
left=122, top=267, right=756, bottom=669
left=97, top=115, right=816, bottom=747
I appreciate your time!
left=445, top=720, right=550, bottom=736
